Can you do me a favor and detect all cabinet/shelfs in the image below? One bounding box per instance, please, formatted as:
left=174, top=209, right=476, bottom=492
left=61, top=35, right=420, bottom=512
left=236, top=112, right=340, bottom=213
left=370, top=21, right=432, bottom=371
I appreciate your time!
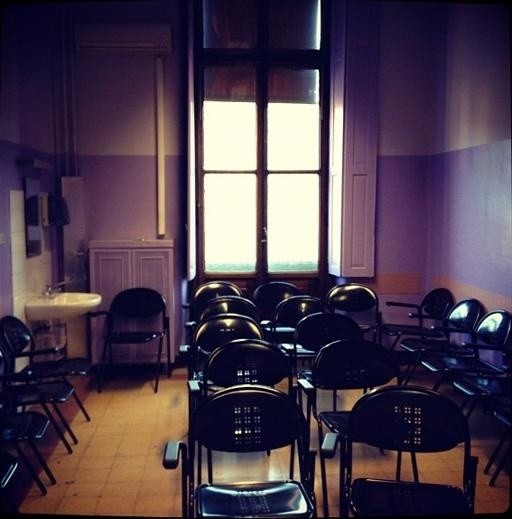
left=88, top=239, right=174, bottom=365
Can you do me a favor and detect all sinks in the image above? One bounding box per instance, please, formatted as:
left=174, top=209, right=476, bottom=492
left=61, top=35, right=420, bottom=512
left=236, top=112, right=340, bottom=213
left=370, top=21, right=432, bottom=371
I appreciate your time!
left=25, top=291, right=102, bottom=322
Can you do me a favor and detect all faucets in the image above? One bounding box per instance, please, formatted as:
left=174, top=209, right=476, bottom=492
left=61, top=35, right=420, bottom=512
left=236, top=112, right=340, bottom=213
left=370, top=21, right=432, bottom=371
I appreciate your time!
left=46, top=281, right=70, bottom=291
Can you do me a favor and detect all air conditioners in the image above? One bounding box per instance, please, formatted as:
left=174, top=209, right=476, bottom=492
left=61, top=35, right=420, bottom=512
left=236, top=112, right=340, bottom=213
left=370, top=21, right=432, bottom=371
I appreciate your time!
left=69, top=19, right=171, bottom=55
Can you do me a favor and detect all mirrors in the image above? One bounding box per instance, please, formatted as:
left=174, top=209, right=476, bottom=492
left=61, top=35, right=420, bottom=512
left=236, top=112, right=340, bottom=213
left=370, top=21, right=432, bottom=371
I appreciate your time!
left=25, top=176, right=42, bottom=257
